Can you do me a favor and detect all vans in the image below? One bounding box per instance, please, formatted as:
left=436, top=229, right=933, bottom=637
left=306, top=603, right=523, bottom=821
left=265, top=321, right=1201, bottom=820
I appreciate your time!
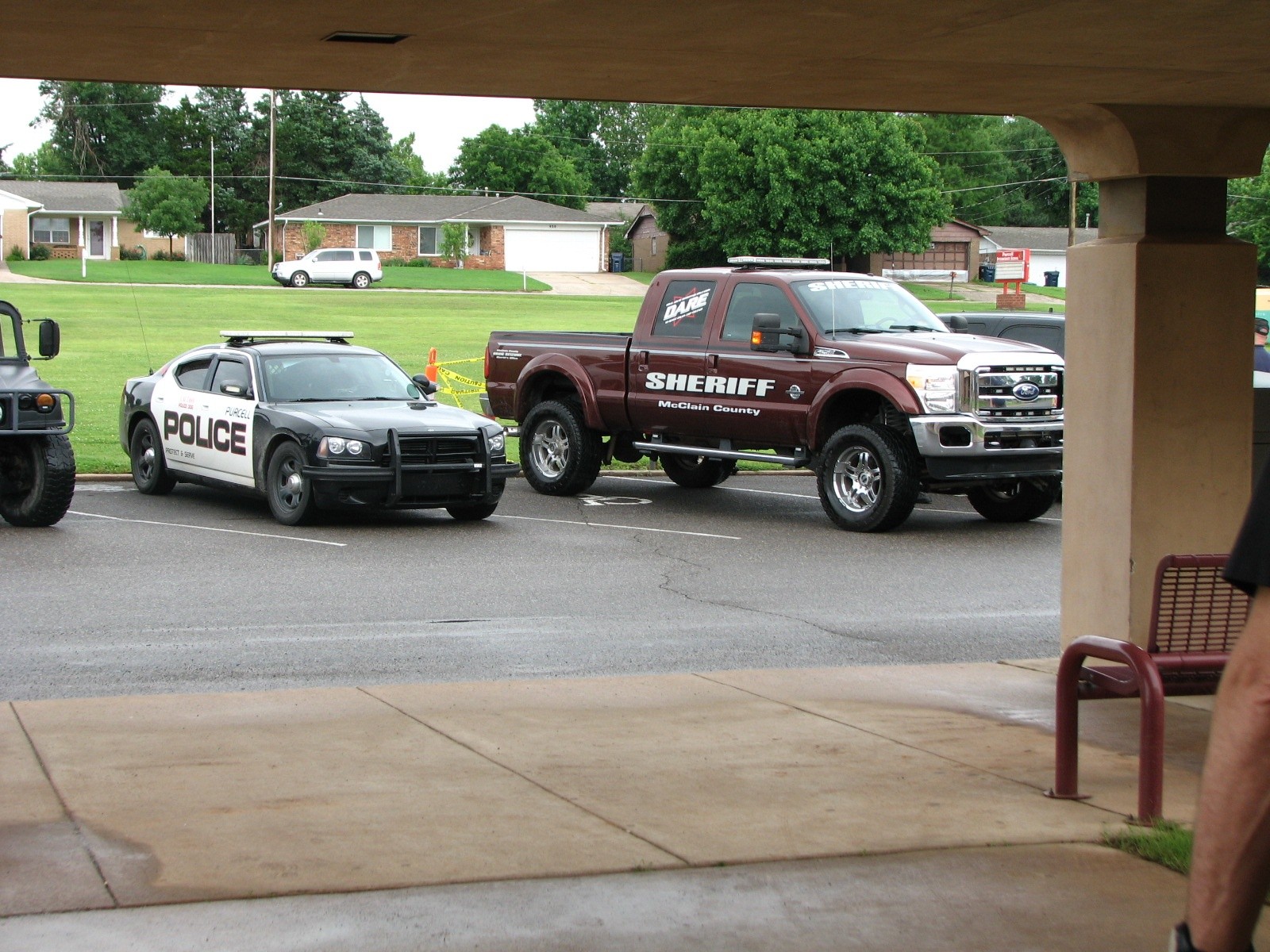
left=272, top=248, right=383, bottom=288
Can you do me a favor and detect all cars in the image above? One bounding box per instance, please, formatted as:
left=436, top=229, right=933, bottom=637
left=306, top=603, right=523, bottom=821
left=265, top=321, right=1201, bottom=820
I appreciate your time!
left=935, top=312, right=1066, bottom=360
left=120, top=331, right=521, bottom=526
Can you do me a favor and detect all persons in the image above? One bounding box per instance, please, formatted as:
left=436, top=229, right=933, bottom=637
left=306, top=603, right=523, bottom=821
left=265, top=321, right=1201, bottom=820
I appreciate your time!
left=1167, top=417, right=1270, bottom=952
left=1253, top=315, right=1270, bottom=373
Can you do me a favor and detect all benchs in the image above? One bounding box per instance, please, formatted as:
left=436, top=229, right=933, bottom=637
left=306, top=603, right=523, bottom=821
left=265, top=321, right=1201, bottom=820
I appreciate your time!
left=1043, top=552, right=1251, bottom=827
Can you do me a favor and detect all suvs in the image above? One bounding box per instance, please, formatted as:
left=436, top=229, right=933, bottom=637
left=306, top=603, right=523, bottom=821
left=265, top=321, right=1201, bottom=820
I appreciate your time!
left=0, top=300, right=76, bottom=528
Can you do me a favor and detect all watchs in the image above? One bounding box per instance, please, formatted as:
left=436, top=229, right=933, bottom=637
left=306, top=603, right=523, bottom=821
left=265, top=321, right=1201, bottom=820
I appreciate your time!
left=1170, top=919, right=1257, bottom=952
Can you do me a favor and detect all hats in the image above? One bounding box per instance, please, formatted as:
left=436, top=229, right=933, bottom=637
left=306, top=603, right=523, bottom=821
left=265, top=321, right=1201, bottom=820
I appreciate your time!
left=1254, top=317, right=1269, bottom=335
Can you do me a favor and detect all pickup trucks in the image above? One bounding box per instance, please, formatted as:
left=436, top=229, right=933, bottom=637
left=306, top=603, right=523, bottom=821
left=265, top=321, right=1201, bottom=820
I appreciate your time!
left=480, top=258, right=1065, bottom=535
